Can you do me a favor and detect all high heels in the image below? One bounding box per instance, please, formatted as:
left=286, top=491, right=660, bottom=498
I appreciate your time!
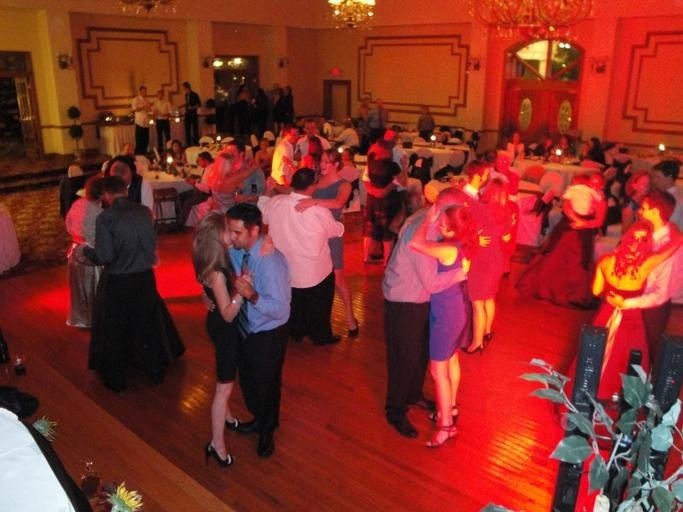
left=206, top=442, right=233, bottom=466
left=462, top=331, right=493, bottom=354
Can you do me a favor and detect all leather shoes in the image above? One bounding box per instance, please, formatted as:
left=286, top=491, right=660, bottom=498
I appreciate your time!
left=289, top=319, right=359, bottom=346
left=388, top=412, right=418, bottom=440
left=225, top=415, right=275, bottom=457
left=410, top=396, right=433, bottom=410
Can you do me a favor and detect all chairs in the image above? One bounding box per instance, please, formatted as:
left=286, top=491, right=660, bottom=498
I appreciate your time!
left=61, top=125, right=634, bottom=254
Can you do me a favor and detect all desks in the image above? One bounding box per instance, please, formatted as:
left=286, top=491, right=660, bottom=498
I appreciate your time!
left=62, top=126, right=682, bottom=257
left=0, top=203, right=22, bottom=363
left=95, top=111, right=167, bottom=160
left=166, top=106, right=215, bottom=150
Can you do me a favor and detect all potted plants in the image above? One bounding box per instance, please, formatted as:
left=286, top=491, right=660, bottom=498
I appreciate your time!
left=65, top=104, right=85, bottom=162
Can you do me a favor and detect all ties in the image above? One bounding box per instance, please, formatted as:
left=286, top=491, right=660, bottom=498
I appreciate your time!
left=236, top=253, right=251, bottom=339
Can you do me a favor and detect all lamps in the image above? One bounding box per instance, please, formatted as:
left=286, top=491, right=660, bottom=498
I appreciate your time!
left=201, top=54, right=247, bottom=68
left=327, top=0, right=378, bottom=28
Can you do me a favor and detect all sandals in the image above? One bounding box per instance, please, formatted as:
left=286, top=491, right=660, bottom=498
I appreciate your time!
left=425, top=404, right=458, bottom=448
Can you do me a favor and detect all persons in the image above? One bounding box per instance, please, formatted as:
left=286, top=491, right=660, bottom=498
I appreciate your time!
left=65, top=77, right=682, bottom=472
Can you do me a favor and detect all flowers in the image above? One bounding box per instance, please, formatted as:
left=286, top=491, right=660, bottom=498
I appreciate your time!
left=101, top=480, right=147, bottom=511
left=31, top=417, right=60, bottom=443
